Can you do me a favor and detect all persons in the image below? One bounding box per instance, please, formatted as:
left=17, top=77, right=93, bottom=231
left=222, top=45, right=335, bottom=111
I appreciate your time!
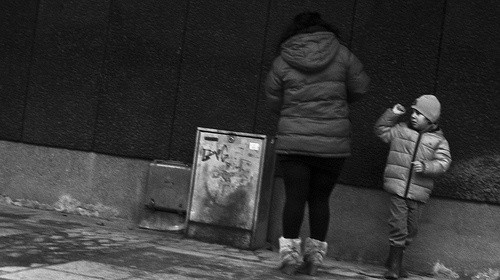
left=375, top=94, right=453, bottom=279
left=263, top=12, right=372, bottom=274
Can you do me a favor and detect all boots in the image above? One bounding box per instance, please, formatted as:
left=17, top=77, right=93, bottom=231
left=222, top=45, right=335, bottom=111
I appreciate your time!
left=279, top=236, right=303, bottom=276
left=303, top=237, right=328, bottom=276
left=384, top=245, right=408, bottom=280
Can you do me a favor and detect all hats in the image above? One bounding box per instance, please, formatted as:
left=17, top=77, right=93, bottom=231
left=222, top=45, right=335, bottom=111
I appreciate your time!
left=411, top=95, right=441, bottom=123
